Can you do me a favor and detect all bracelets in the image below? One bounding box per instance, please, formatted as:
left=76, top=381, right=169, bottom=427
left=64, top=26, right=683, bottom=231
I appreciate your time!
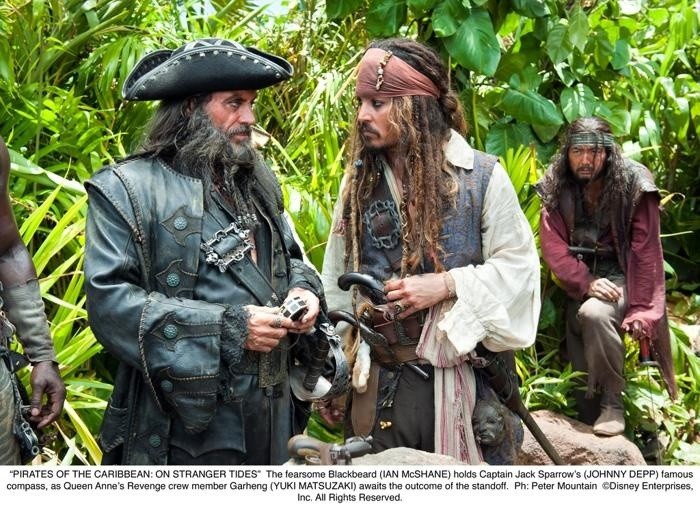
left=442, top=271, right=457, bottom=299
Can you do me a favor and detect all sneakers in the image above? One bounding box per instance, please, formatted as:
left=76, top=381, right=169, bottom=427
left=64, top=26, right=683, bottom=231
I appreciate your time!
left=593, top=399, right=626, bottom=436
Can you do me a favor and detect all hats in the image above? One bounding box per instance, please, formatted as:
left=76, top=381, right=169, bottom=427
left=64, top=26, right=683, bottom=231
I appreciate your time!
left=121, top=38, right=295, bottom=101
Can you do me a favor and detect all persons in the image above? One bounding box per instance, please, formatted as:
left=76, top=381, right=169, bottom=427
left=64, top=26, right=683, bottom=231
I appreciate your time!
left=0, top=133, right=68, bottom=465
left=79, top=39, right=330, bottom=465
left=307, top=37, right=543, bottom=465
left=531, top=117, right=677, bottom=438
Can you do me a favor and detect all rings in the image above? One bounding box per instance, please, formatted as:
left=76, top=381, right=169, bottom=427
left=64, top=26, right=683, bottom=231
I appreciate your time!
left=274, top=315, right=282, bottom=328
left=394, top=300, right=406, bottom=312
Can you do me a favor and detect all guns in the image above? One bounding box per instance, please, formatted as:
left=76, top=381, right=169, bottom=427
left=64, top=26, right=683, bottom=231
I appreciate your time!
left=327, top=311, right=429, bottom=381
left=289, top=434, right=373, bottom=467
left=19, top=422, right=39, bottom=465
left=337, top=271, right=567, bottom=465
